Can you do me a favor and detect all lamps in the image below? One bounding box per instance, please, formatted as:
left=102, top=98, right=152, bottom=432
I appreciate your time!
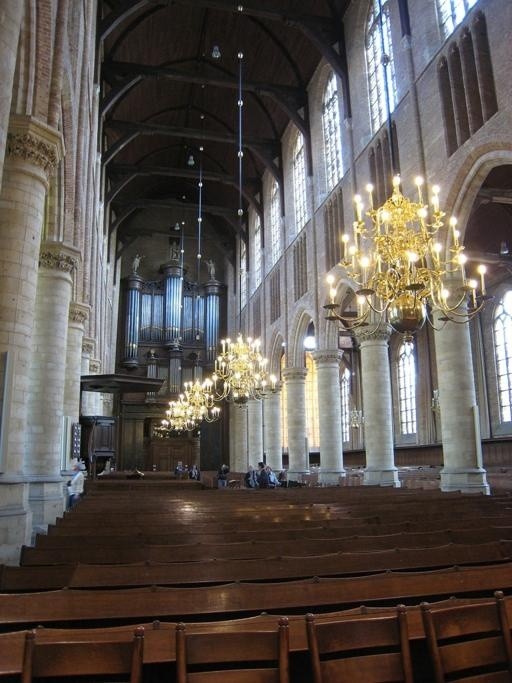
left=350, top=403, right=364, bottom=430
left=321, top=0, right=496, bottom=351
left=150, top=0, right=285, bottom=444
left=430, top=388, right=441, bottom=416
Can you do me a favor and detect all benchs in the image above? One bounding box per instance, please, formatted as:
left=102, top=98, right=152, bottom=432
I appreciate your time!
left=0, top=457, right=512, bottom=683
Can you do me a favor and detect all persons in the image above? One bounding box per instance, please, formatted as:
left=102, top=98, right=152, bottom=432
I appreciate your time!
left=171, top=238, right=182, bottom=258
left=175, top=461, right=287, bottom=490
left=65, top=460, right=85, bottom=509
left=205, top=259, right=218, bottom=278
left=133, top=252, right=147, bottom=274
